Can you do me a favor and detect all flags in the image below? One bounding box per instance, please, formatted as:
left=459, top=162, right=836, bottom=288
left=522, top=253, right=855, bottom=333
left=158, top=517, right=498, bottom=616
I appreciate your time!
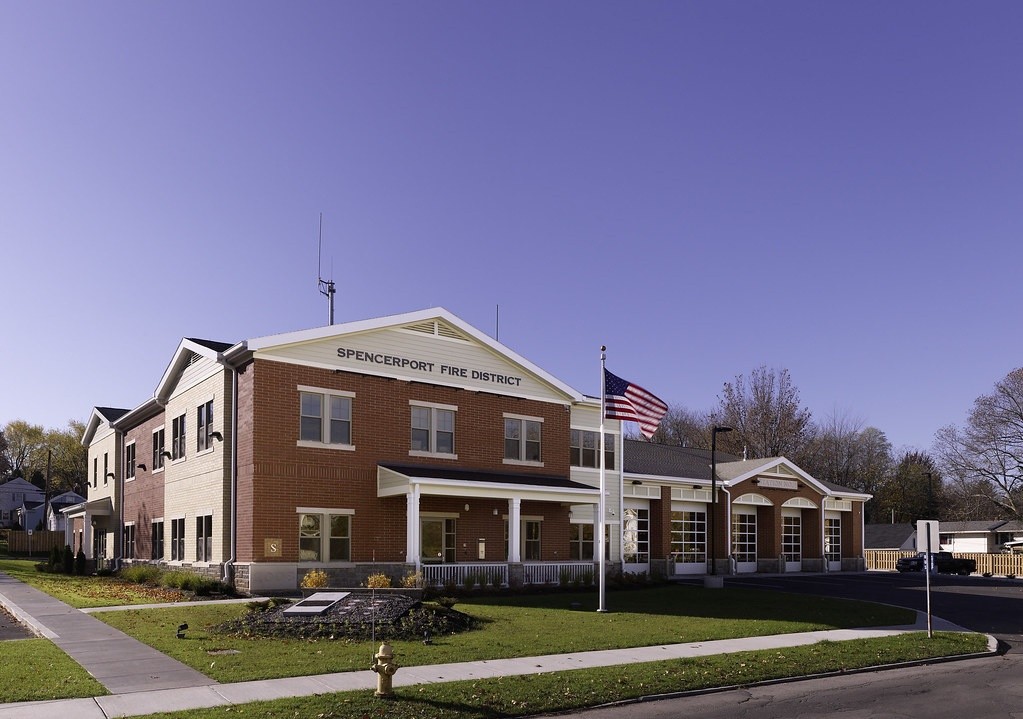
left=604, top=367, right=668, bottom=443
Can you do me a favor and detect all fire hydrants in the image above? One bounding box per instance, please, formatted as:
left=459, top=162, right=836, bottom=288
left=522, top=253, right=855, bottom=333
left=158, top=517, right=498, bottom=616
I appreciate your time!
left=371, top=640, right=399, bottom=698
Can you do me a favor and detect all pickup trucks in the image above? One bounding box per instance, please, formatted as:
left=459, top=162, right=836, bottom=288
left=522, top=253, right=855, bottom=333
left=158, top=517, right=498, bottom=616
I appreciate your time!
left=896, top=551, right=977, bottom=577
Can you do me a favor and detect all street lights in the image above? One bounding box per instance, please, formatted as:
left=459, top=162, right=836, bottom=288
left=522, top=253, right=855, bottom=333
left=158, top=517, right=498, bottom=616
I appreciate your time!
left=922, top=471, right=931, bottom=520
left=709, top=427, right=734, bottom=575
